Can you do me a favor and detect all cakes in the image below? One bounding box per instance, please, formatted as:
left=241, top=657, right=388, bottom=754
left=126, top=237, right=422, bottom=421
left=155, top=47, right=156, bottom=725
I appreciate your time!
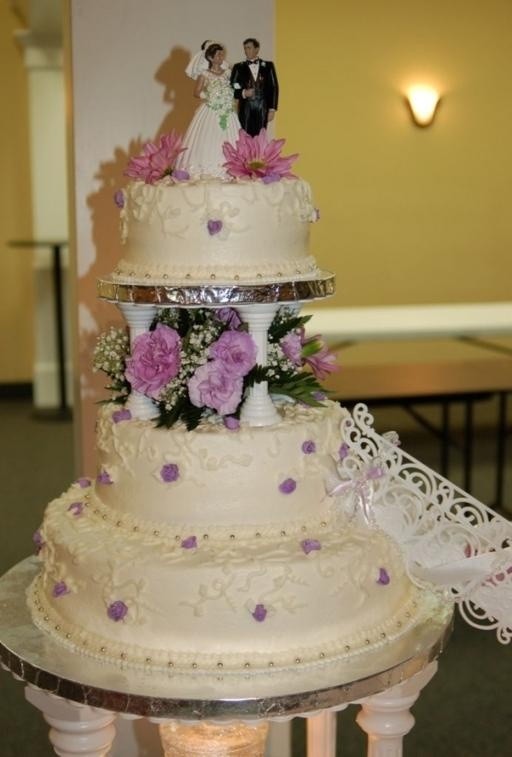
left=26, top=37, right=426, bottom=670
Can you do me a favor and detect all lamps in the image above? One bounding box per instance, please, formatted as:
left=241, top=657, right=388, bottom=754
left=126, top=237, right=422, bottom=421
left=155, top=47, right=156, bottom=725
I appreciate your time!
left=399, top=85, right=442, bottom=125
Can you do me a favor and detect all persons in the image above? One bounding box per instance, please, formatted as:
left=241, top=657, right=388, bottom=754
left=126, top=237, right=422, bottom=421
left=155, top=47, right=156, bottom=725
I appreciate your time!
left=172, top=41, right=242, bottom=176
left=229, top=38, right=280, bottom=136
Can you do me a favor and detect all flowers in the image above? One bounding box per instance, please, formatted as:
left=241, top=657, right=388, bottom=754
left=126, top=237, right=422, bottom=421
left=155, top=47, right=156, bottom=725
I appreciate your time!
left=218, top=125, right=302, bottom=184
left=116, top=130, right=194, bottom=185
left=91, top=305, right=343, bottom=432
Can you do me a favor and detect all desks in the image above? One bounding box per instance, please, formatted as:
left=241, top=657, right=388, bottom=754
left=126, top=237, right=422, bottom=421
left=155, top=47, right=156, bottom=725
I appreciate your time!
left=291, top=331, right=512, bottom=524
left=1, top=542, right=457, bottom=756
left=454, top=328, right=512, bottom=510
